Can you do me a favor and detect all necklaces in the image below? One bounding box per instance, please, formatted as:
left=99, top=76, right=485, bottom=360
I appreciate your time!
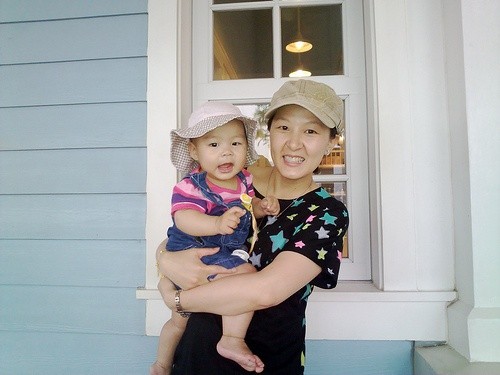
left=266, top=166, right=313, bottom=217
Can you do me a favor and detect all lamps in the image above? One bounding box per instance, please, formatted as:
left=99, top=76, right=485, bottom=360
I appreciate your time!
left=285, top=8, right=313, bottom=77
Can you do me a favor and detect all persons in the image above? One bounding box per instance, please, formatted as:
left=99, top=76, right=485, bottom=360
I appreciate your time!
left=155, top=79, right=349, bottom=375
left=151, top=101, right=280, bottom=375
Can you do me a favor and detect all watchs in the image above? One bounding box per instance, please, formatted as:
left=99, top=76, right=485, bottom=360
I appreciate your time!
left=175, top=290, right=191, bottom=317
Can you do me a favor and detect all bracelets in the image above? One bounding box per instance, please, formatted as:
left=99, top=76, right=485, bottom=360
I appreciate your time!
left=157, top=249, right=166, bottom=279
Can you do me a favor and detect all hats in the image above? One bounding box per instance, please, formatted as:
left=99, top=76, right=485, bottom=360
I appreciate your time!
left=264, top=79, right=345, bottom=135
left=169, top=101, right=261, bottom=171
